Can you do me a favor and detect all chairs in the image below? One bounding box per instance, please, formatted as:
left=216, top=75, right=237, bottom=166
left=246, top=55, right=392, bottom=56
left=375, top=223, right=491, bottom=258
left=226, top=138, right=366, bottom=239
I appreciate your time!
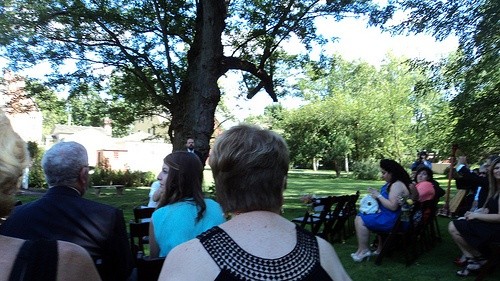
left=371, top=187, right=443, bottom=267
left=95, top=207, right=167, bottom=281
left=292, top=189, right=360, bottom=245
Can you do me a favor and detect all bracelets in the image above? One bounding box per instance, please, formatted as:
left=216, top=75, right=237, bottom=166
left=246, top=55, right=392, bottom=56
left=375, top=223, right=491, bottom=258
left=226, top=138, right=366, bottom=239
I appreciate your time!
left=378, top=194, right=382, bottom=198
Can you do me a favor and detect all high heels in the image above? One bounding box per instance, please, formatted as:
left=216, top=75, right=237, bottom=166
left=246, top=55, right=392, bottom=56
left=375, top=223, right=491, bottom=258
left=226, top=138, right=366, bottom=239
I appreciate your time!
left=474, top=255, right=489, bottom=266
left=456, top=257, right=482, bottom=276
left=350, top=247, right=371, bottom=262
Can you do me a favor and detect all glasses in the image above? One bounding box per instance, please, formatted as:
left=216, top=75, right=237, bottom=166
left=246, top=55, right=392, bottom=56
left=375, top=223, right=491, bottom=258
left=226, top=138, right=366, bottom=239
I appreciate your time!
left=78, top=164, right=96, bottom=175
left=478, top=172, right=488, bottom=175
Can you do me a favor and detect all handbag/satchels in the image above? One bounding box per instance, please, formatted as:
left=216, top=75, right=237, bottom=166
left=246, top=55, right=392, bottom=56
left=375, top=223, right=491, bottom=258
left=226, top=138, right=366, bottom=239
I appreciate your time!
left=359, top=195, right=380, bottom=215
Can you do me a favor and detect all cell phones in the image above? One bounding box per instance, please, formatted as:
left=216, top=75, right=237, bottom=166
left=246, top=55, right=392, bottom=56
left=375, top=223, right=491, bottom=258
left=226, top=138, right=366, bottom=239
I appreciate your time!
left=369, top=187, right=373, bottom=190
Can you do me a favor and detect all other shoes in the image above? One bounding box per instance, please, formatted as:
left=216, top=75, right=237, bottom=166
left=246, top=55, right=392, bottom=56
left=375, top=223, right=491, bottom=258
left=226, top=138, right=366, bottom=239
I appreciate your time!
left=454, top=258, right=465, bottom=264
left=373, top=247, right=383, bottom=254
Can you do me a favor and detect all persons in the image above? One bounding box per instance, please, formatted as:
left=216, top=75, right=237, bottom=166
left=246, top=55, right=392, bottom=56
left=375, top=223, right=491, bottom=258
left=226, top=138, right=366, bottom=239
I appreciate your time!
left=0, top=108, right=101, bottom=281
left=142, top=181, right=161, bottom=239
left=186, top=138, right=205, bottom=166
left=351, top=152, right=445, bottom=262
left=449, top=156, right=500, bottom=276
left=158, top=124, right=353, bottom=281
left=149, top=152, right=226, bottom=257
left=0, top=142, right=133, bottom=281
